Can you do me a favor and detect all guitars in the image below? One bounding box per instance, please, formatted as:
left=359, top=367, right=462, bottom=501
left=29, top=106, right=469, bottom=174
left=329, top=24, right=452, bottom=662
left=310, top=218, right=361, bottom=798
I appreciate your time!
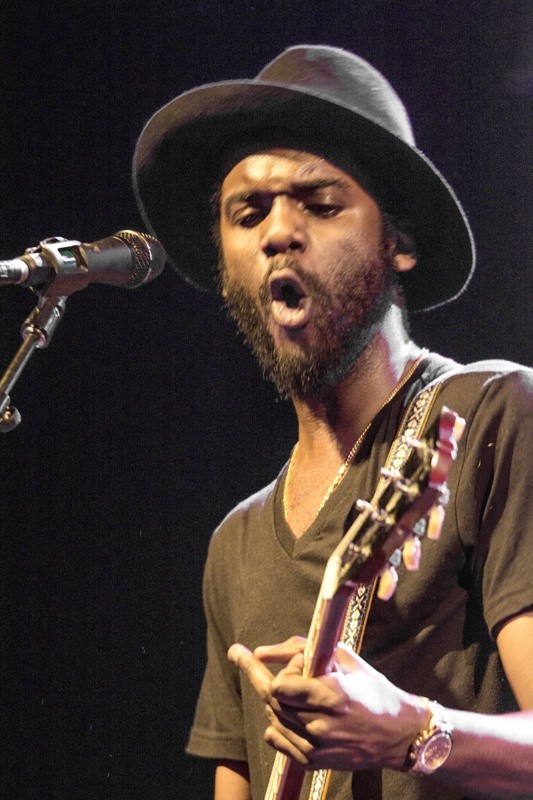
left=263, top=405, right=466, bottom=800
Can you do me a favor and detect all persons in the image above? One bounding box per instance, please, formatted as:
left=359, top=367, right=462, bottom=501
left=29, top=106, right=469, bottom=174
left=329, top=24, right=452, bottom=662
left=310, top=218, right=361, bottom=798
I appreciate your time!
left=129, top=41, right=533, bottom=800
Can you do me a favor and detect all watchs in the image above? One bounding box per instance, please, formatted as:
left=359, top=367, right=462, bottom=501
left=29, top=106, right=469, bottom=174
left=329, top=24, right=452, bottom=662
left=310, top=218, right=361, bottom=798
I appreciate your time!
left=390, top=692, right=455, bottom=779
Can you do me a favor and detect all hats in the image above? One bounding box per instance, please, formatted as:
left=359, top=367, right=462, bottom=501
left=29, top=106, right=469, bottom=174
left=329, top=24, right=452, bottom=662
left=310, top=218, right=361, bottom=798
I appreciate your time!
left=131, top=45, right=475, bottom=313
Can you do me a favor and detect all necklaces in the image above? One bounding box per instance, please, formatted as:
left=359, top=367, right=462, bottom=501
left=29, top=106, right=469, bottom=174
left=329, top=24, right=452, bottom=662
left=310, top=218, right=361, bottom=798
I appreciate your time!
left=282, top=347, right=428, bottom=528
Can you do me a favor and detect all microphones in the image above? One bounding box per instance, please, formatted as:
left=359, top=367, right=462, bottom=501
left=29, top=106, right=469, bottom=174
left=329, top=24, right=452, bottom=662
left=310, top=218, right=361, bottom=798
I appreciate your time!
left=1, top=227, right=168, bottom=293
